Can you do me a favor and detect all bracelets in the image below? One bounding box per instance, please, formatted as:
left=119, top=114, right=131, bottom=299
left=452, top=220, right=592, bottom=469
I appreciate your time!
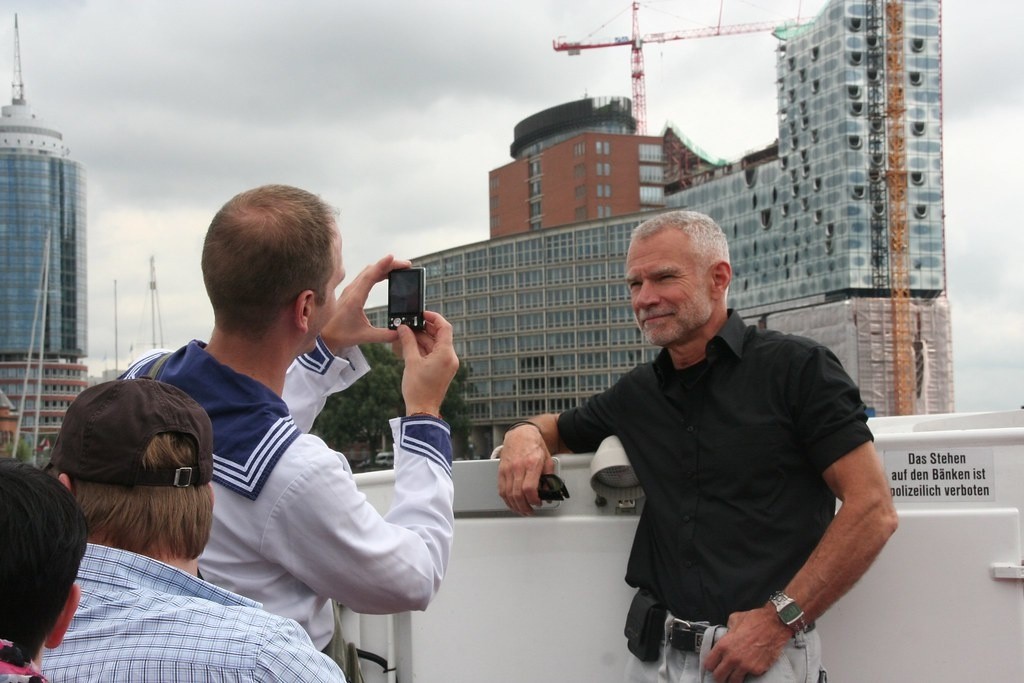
left=503, top=421, right=545, bottom=440
left=406, top=412, right=442, bottom=419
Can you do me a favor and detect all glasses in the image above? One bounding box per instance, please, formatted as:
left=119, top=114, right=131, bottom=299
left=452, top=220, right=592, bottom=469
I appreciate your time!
left=537, top=472, right=570, bottom=504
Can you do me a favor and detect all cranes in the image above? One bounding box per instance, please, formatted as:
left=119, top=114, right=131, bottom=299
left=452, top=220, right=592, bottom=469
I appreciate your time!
left=551, top=0, right=790, bottom=133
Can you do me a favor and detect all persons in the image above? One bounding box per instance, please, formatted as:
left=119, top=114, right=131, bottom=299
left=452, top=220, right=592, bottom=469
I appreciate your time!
left=1, top=455, right=93, bottom=682
left=37, top=378, right=355, bottom=683
left=496, top=209, right=900, bottom=683
left=111, top=183, right=462, bottom=683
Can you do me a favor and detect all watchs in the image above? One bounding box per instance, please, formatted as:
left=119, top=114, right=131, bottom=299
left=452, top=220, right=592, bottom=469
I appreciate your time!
left=769, top=590, right=809, bottom=636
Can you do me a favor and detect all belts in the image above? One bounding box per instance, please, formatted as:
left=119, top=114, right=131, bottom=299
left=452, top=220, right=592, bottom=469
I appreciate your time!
left=660, top=616, right=816, bottom=656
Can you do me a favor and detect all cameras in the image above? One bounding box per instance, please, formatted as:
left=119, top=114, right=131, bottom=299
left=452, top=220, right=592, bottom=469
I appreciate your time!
left=388, top=267, right=426, bottom=331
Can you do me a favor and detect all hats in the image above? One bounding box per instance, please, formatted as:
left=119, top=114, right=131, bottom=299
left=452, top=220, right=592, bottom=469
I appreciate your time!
left=38, top=375, right=214, bottom=489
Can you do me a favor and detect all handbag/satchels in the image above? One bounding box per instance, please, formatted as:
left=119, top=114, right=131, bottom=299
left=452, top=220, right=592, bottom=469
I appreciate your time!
left=320, top=597, right=367, bottom=683
